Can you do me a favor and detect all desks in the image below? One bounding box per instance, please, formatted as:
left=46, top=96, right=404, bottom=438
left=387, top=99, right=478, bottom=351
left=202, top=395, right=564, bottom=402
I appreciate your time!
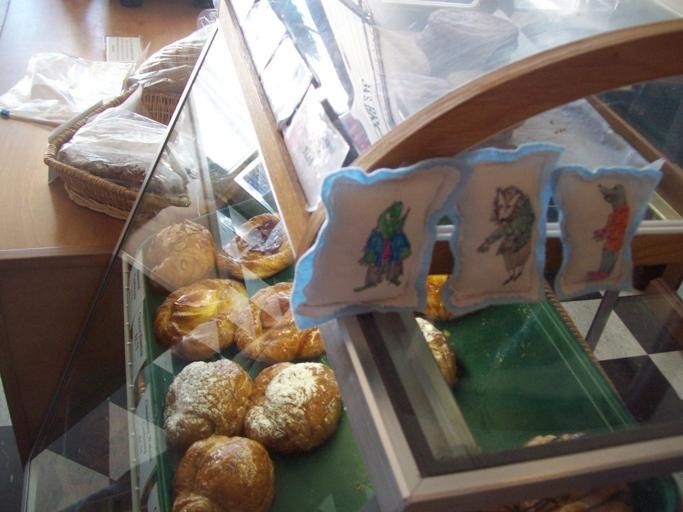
left=0, top=0, right=214, bottom=469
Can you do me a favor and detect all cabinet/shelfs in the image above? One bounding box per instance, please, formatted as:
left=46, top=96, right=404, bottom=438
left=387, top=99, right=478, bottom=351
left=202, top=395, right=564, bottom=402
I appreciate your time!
left=18, top=0, right=683, bottom=512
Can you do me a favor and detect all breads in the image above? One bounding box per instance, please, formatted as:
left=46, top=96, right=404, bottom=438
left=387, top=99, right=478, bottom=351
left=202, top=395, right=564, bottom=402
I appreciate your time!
left=214, top=210, right=295, bottom=282
left=419, top=274, right=461, bottom=323
left=243, top=359, right=344, bottom=454
left=232, top=280, right=326, bottom=363
left=139, top=218, right=218, bottom=293
left=150, top=277, right=251, bottom=359
left=169, top=435, right=279, bottom=511
left=159, top=358, right=254, bottom=455
left=414, top=315, right=457, bottom=391
